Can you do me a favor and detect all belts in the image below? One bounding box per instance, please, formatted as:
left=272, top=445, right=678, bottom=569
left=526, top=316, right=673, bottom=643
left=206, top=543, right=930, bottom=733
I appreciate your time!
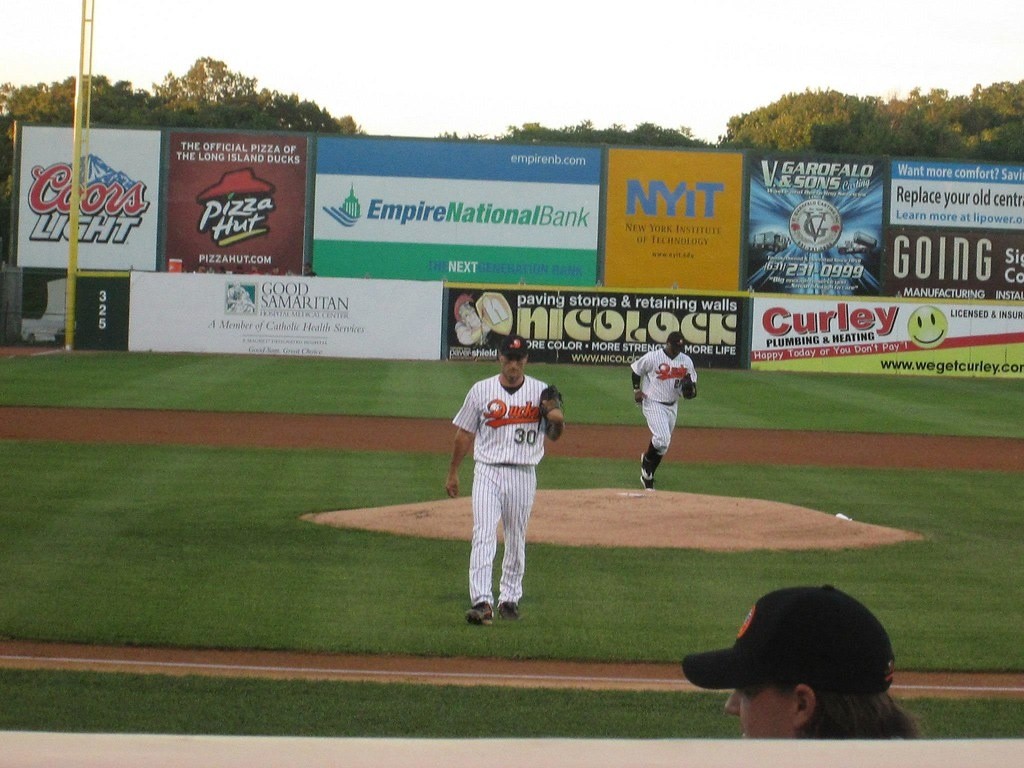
left=661, top=401, right=674, bottom=407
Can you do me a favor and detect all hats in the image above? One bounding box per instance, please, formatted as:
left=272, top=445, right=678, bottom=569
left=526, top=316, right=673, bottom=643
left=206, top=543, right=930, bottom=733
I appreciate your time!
left=499, top=335, right=528, bottom=357
left=683, top=585, right=893, bottom=695
left=669, top=332, right=685, bottom=346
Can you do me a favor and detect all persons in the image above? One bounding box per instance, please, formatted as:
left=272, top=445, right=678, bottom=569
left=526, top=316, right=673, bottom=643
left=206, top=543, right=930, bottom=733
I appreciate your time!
left=678, top=583, right=921, bottom=742
left=630, top=332, right=699, bottom=490
left=443, top=334, right=564, bottom=626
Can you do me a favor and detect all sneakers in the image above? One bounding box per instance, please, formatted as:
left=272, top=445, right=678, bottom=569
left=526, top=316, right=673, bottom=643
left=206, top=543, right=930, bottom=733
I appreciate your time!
left=639, top=476, right=655, bottom=492
left=640, top=453, right=653, bottom=481
left=499, top=603, right=519, bottom=621
left=464, top=603, right=494, bottom=626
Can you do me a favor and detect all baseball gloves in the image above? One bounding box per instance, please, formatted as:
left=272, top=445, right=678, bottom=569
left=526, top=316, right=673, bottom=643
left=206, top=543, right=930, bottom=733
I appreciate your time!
left=538, top=384, right=566, bottom=418
left=681, top=372, right=697, bottom=400
